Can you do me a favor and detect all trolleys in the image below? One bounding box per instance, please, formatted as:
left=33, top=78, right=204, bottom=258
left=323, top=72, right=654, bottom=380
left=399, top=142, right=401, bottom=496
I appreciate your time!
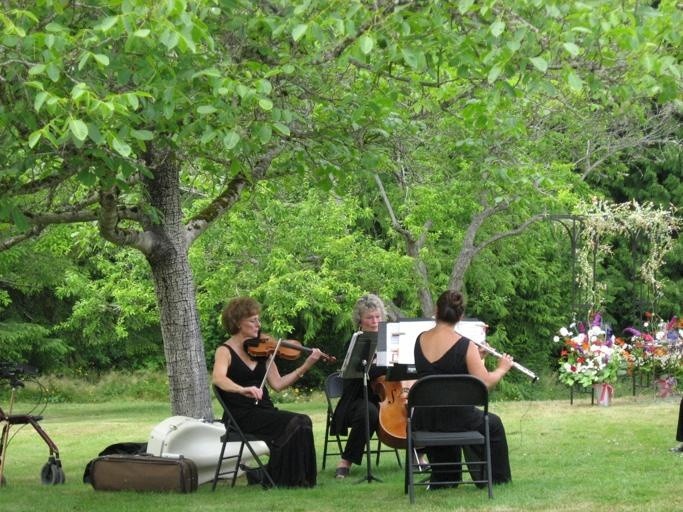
left=0, top=361, right=65, bottom=487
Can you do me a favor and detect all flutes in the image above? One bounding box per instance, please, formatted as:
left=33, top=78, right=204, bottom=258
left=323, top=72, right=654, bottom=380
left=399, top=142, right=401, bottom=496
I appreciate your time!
left=431, top=316, right=540, bottom=383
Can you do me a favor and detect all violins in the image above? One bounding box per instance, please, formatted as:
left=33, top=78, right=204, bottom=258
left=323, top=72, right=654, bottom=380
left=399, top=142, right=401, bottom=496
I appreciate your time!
left=248, top=332, right=337, bottom=365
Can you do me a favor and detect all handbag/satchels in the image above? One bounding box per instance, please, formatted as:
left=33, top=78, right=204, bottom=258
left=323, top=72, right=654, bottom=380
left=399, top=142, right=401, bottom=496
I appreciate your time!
left=239, top=462, right=267, bottom=485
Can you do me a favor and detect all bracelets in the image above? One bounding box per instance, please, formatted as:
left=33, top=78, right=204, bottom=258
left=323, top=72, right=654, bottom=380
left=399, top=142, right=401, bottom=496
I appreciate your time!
left=296, top=368, right=303, bottom=378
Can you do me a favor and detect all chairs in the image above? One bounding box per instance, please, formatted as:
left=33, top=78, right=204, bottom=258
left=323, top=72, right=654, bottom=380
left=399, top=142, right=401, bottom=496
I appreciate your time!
left=212, top=371, right=494, bottom=505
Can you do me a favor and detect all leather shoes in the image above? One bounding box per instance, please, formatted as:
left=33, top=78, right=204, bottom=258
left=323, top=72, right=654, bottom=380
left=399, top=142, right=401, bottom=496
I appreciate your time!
left=335, top=467, right=349, bottom=478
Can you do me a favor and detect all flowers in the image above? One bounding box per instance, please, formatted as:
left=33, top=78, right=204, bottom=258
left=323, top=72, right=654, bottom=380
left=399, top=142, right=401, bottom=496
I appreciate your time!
left=553, top=311, right=682, bottom=389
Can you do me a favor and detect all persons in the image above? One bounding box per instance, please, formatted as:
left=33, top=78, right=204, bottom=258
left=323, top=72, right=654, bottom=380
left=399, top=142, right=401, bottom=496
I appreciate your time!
left=212, top=296, right=322, bottom=489
left=414, top=289, right=514, bottom=489
left=668, top=396, right=683, bottom=452
left=330, top=295, right=431, bottom=479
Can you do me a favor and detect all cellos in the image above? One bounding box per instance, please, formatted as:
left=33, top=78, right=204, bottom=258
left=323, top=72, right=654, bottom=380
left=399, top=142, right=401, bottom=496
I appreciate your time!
left=370, top=375, right=420, bottom=448
